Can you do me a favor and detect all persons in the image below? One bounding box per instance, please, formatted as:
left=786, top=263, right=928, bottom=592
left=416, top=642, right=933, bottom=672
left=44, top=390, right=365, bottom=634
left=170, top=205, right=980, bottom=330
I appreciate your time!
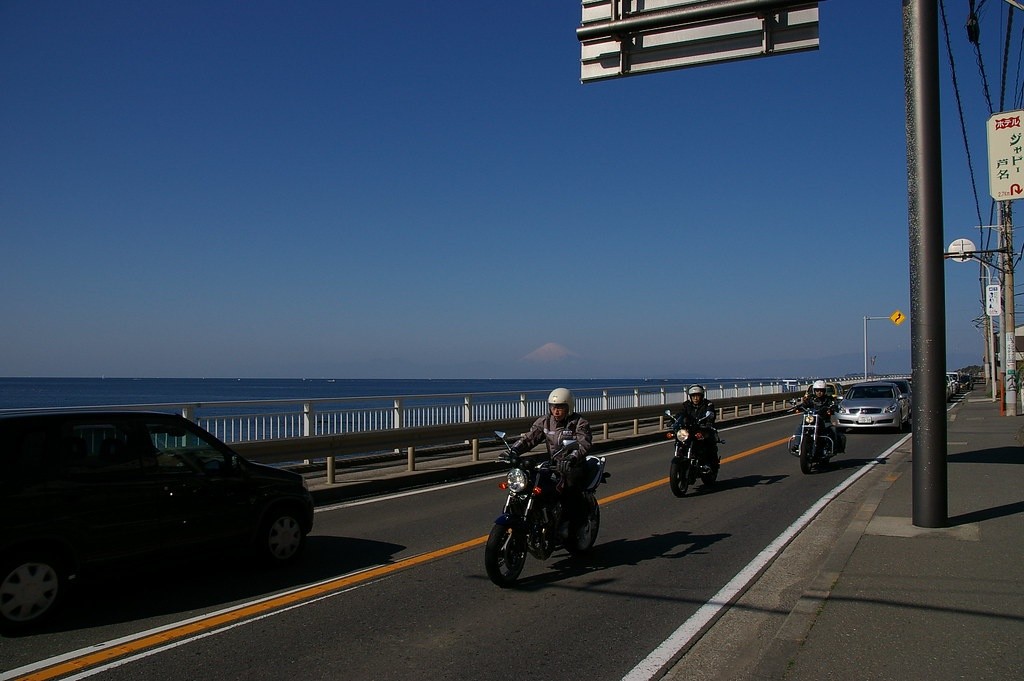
left=670, top=385, right=716, bottom=463
left=791, top=380, right=837, bottom=451
left=499, top=388, right=593, bottom=492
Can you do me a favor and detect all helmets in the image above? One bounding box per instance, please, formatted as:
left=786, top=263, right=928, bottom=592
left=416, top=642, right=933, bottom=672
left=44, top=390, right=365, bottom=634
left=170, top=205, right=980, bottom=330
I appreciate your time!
left=547, top=388, right=575, bottom=415
left=688, top=384, right=705, bottom=401
left=813, top=380, right=826, bottom=396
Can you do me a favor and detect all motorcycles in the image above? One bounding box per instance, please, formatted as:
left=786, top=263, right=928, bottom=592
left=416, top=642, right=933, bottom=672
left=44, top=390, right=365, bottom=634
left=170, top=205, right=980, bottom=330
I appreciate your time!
left=485, top=431, right=610, bottom=588
left=787, top=399, right=847, bottom=474
left=665, top=409, right=725, bottom=498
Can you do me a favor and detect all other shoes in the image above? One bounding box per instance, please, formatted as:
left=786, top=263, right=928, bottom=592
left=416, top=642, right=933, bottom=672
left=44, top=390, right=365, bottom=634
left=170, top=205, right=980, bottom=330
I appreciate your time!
left=792, top=444, right=798, bottom=451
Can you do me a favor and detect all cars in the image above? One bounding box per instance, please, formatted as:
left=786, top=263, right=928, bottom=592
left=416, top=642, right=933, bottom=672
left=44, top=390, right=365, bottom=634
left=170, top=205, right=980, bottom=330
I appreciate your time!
left=804, top=382, right=843, bottom=414
left=876, top=371, right=960, bottom=416
left=0, top=410, right=315, bottom=632
left=831, top=382, right=908, bottom=433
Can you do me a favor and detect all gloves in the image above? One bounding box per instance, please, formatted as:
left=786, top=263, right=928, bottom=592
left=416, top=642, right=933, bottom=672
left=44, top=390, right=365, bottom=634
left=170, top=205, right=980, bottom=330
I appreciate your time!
left=499, top=447, right=518, bottom=463
left=560, top=454, right=576, bottom=472
left=702, top=418, right=712, bottom=429
